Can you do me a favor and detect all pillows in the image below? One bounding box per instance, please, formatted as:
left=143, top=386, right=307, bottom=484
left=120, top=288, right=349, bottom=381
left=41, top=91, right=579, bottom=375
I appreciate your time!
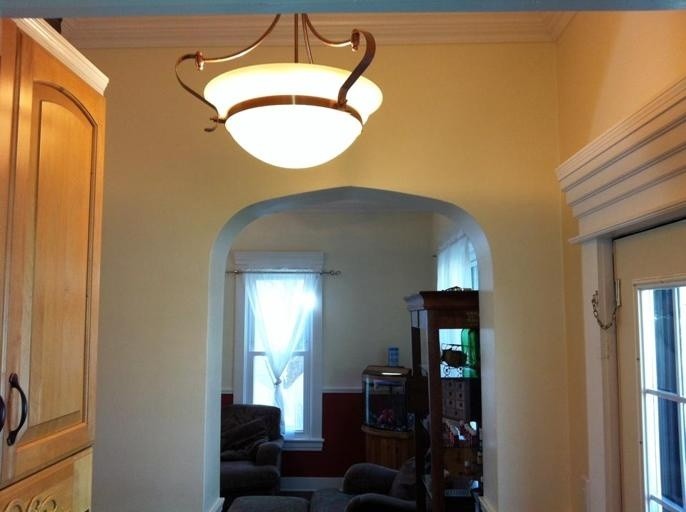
left=221, top=417, right=269, bottom=461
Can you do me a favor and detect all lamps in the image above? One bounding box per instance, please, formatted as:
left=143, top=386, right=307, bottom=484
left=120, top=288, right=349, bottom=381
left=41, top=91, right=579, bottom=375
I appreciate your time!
left=175, top=12, right=384, bottom=170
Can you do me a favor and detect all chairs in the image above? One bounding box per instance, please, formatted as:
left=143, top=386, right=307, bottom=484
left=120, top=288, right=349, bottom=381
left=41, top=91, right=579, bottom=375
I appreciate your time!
left=310, top=456, right=417, bottom=512
left=220, top=404, right=284, bottom=497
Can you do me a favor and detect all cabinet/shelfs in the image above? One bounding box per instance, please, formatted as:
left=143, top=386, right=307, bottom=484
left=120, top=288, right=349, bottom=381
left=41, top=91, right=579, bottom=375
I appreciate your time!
left=403, top=290, right=483, bottom=512
left=0, top=18, right=111, bottom=512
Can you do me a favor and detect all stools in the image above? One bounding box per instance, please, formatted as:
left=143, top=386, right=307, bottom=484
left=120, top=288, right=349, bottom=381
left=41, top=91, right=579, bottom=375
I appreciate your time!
left=226, top=495, right=310, bottom=512
